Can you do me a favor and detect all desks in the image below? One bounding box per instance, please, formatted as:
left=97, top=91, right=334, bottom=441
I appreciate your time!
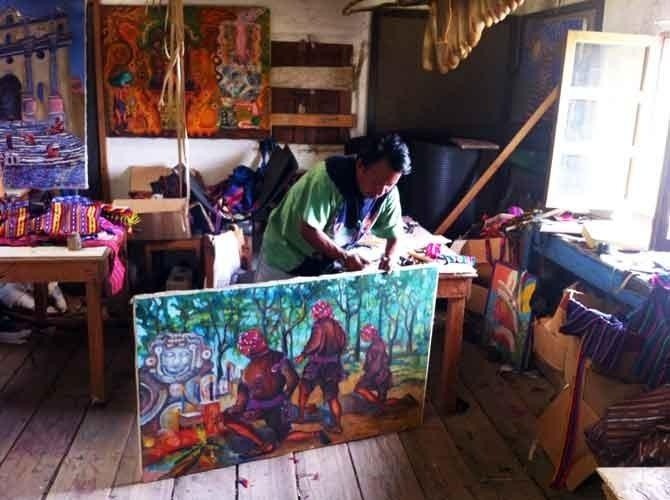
left=0, top=228, right=129, bottom=407
left=519, top=220, right=648, bottom=309
left=398, top=214, right=480, bottom=414
left=145, top=226, right=201, bottom=279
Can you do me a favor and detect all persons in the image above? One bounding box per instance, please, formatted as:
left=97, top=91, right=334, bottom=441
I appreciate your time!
left=252, top=129, right=414, bottom=282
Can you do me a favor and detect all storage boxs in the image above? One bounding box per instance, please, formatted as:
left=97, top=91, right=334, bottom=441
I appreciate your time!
left=529, top=281, right=670, bottom=500
left=110, top=197, right=191, bottom=241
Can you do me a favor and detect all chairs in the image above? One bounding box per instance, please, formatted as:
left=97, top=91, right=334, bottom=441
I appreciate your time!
left=204, top=224, right=242, bottom=288
